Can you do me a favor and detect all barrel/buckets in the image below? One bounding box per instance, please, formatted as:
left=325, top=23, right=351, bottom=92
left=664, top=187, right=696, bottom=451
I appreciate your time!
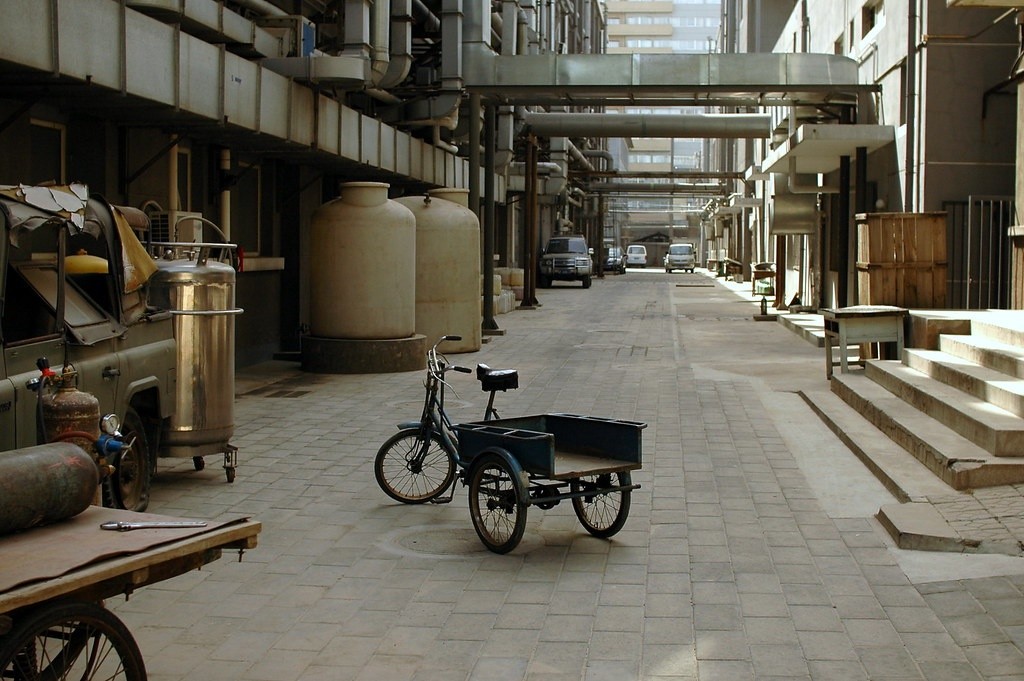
left=481, top=267, right=524, bottom=316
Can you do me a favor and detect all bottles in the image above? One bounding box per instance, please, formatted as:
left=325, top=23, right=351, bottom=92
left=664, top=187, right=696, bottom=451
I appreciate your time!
left=309, top=182, right=416, bottom=339
left=392, top=188, right=482, bottom=354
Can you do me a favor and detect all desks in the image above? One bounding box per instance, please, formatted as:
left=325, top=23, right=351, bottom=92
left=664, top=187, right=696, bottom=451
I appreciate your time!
left=751, top=268, right=774, bottom=297
left=818, top=304, right=908, bottom=381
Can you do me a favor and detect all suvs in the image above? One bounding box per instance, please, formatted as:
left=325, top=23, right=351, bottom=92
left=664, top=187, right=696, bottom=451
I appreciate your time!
left=539, top=235, right=594, bottom=290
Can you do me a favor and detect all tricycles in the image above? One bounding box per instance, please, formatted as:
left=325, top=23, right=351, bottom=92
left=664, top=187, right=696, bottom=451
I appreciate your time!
left=375, top=336, right=649, bottom=553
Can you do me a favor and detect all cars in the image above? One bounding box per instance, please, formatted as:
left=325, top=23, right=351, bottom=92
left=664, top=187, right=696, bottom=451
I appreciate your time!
left=601, top=247, right=630, bottom=274
left=663, top=253, right=670, bottom=273
left=626, top=245, right=647, bottom=268
left=664, top=244, right=696, bottom=274
